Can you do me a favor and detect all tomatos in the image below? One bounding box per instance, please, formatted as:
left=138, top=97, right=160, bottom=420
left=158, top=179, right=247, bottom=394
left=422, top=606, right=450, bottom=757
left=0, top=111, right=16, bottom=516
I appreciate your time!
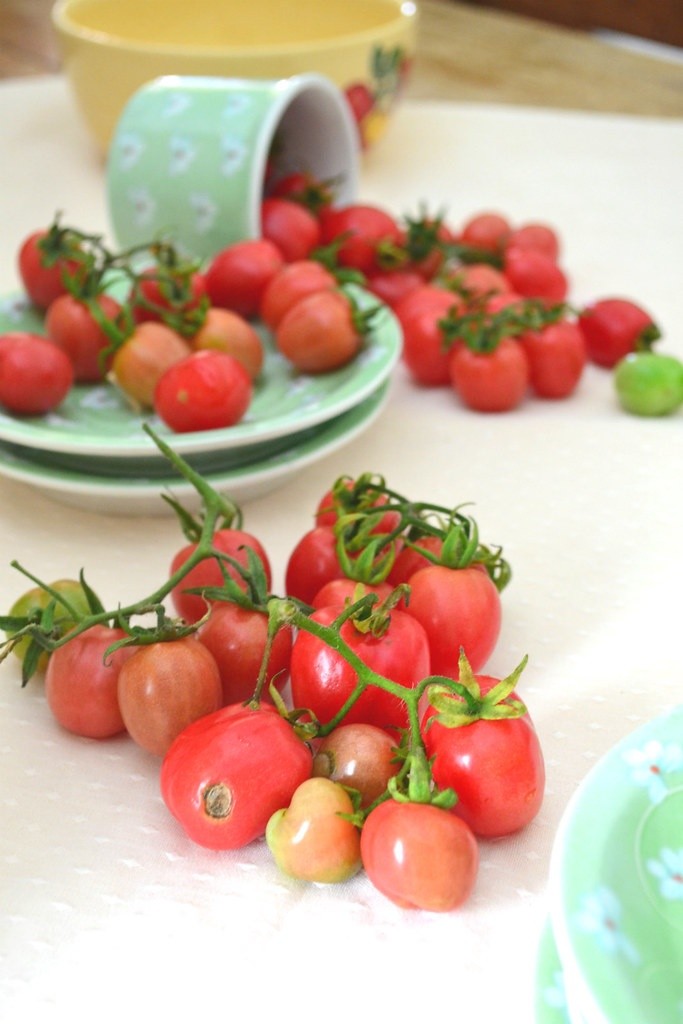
left=0, top=141, right=682, bottom=432
left=1, top=424, right=546, bottom=915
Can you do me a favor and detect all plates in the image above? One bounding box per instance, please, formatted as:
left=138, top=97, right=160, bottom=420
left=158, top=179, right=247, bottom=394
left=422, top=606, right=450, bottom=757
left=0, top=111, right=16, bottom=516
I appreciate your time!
left=0, top=268, right=402, bottom=457
left=0, top=377, right=392, bottom=516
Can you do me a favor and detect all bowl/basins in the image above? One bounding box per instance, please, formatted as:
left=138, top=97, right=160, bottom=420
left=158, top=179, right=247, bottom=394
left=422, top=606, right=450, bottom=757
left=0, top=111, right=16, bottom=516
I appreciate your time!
left=52, top=0, right=413, bottom=170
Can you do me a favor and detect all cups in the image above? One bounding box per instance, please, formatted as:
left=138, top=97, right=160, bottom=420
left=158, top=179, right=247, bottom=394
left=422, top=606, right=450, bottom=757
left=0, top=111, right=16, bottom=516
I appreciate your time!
left=106, top=71, right=362, bottom=266
